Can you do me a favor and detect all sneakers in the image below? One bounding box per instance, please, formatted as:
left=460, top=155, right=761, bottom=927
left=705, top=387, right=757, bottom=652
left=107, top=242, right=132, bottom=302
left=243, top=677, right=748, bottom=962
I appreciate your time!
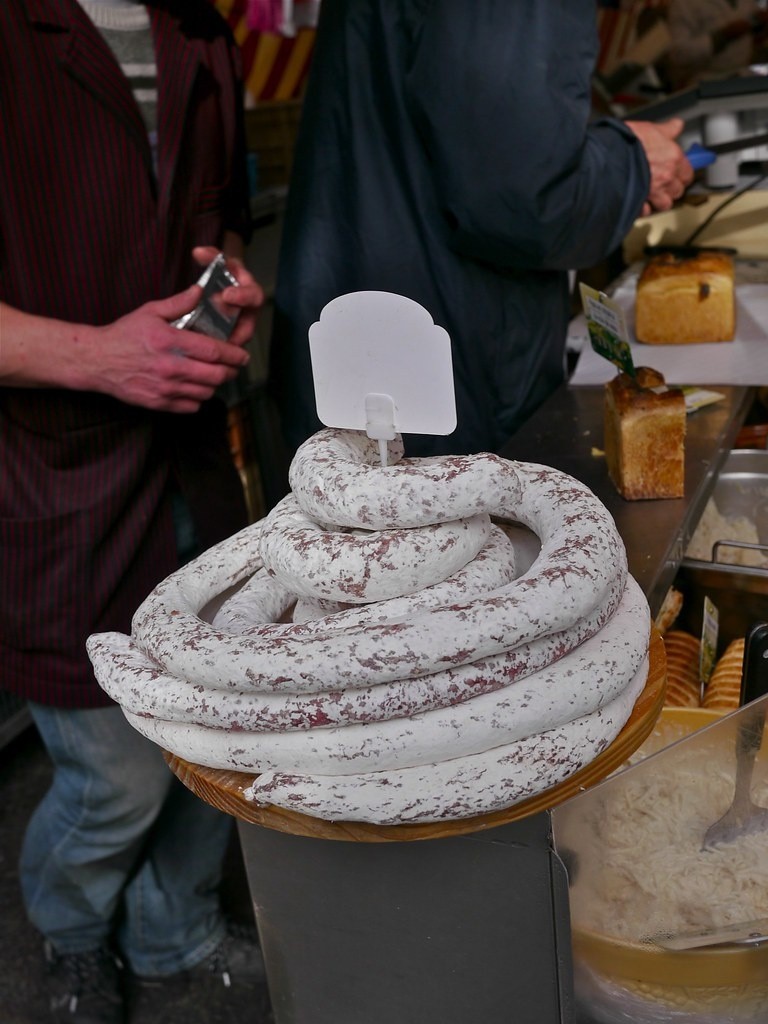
left=39, top=935, right=128, bottom=1024
left=131, top=923, right=271, bottom=990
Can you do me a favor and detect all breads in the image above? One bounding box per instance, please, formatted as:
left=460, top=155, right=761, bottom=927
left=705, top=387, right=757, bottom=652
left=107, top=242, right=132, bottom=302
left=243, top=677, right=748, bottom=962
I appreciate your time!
left=660, top=629, right=751, bottom=713
left=602, top=365, right=688, bottom=500
left=636, top=245, right=738, bottom=343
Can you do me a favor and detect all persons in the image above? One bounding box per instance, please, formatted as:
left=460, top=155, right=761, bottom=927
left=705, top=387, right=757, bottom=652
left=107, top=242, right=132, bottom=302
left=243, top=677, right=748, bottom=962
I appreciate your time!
left=273, top=0, right=694, bottom=502
left=0, top=0, right=266, bottom=1024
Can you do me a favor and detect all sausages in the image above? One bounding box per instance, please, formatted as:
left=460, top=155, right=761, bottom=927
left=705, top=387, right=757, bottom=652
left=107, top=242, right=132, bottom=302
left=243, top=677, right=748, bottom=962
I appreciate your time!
left=86, top=423, right=650, bottom=824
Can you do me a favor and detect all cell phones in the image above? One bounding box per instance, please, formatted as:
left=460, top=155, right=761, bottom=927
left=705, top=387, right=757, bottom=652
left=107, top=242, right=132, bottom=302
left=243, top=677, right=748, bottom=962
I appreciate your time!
left=170, top=260, right=241, bottom=341
